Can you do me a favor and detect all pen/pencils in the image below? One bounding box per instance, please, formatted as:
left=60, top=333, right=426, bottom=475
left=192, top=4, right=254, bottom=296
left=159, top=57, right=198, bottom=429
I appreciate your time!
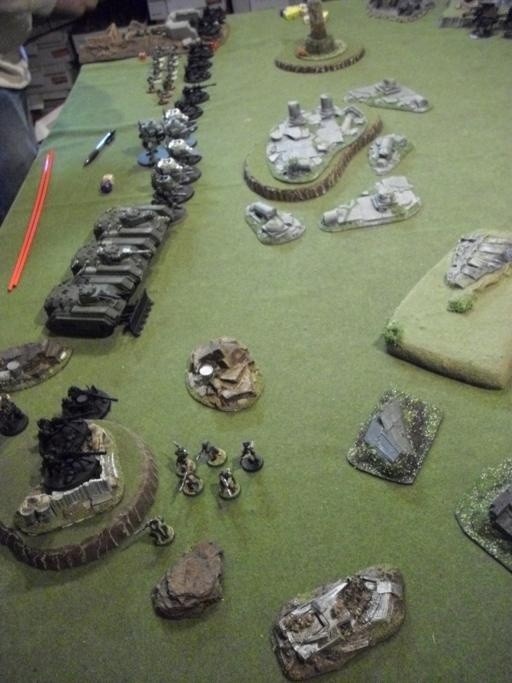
left=83, top=128, right=116, bottom=167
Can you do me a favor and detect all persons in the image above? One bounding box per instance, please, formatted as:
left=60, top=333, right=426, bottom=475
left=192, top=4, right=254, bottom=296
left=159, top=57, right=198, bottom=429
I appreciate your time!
left=0, top=0, right=100, bottom=233
